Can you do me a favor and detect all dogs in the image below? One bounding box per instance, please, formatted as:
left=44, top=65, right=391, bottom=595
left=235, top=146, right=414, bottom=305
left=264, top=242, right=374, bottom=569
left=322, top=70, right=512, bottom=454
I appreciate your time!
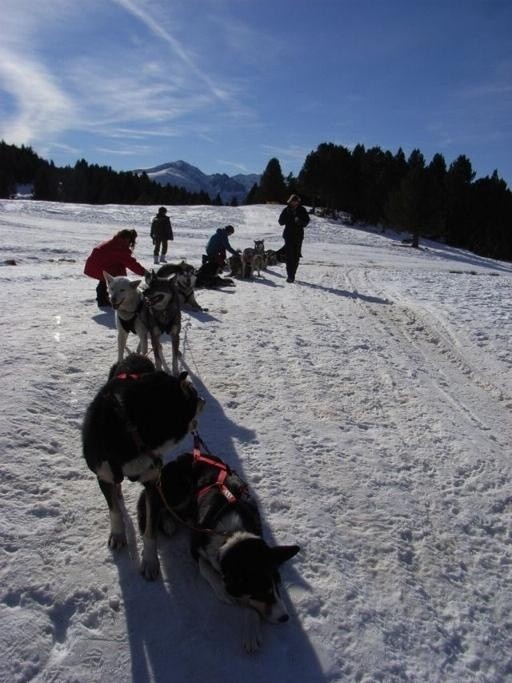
left=102, top=260, right=202, bottom=376
left=80, top=348, right=207, bottom=582
left=136, top=451, right=302, bottom=655
left=195, top=239, right=277, bottom=290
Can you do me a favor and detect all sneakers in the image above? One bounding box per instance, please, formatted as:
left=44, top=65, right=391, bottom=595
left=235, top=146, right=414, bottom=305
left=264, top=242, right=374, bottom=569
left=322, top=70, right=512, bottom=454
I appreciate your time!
left=286, top=275, right=295, bottom=282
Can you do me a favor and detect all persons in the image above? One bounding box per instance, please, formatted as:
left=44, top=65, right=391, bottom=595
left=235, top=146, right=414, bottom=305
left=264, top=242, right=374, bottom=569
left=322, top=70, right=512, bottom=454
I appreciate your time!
left=83, top=228, right=151, bottom=309
left=149, top=205, right=175, bottom=265
left=276, top=193, right=310, bottom=283
left=204, top=224, right=242, bottom=290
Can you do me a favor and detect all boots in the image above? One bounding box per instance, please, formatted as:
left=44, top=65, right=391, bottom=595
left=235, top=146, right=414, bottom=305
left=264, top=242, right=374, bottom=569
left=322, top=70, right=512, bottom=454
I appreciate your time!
left=154, top=254, right=167, bottom=265
